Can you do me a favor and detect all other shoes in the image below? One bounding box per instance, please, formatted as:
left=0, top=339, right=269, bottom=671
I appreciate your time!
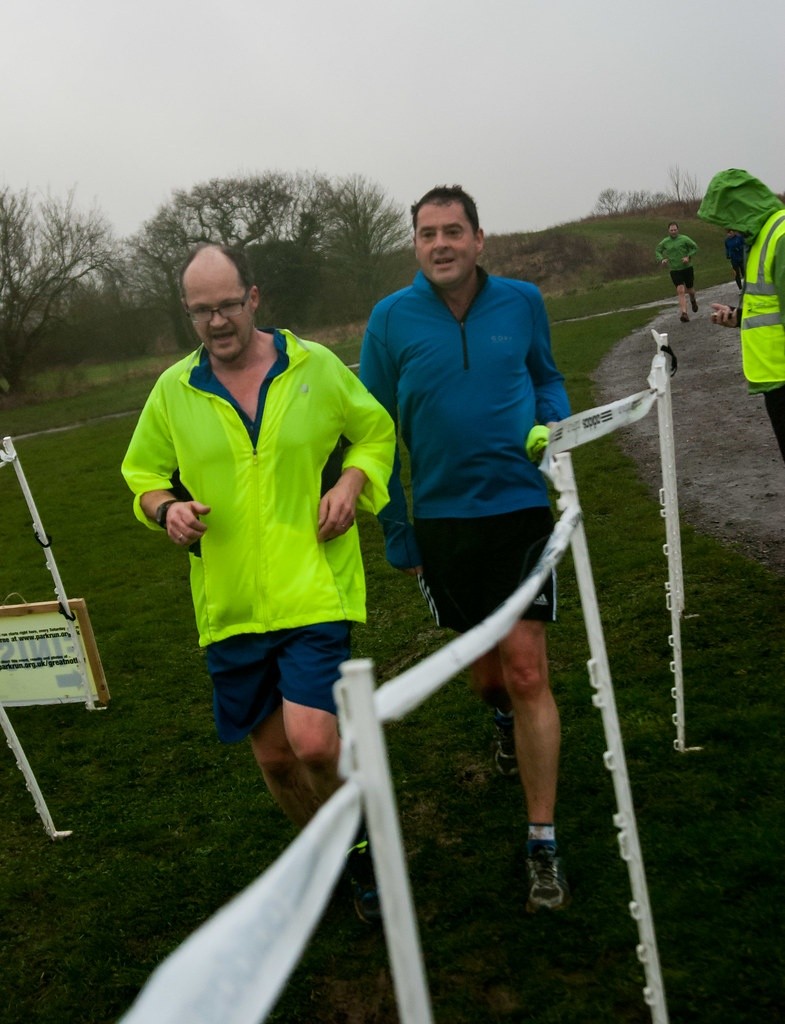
left=691, top=299, right=698, bottom=313
left=739, top=289, right=743, bottom=295
left=680, top=314, right=689, bottom=322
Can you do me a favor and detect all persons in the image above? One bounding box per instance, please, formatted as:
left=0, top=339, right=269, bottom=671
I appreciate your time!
left=357, top=186, right=575, bottom=911
left=697, top=169, right=785, bottom=461
left=656, top=222, right=698, bottom=321
left=121, top=243, right=399, bottom=921
left=725, top=230, right=745, bottom=295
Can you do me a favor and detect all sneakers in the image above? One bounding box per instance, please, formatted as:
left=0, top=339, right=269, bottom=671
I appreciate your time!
left=494, top=709, right=520, bottom=777
left=345, top=845, right=382, bottom=925
left=526, top=846, right=569, bottom=916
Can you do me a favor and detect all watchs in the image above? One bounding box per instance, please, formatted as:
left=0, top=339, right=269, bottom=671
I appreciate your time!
left=156, top=499, right=177, bottom=529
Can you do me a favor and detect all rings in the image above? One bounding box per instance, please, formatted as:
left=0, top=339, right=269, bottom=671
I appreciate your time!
left=341, top=524, right=347, bottom=528
left=178, top=534, right=182, bottom=539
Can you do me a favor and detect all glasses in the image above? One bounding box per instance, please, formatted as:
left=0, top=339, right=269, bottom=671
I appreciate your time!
left=184, top=287, right=251, bottom=324
